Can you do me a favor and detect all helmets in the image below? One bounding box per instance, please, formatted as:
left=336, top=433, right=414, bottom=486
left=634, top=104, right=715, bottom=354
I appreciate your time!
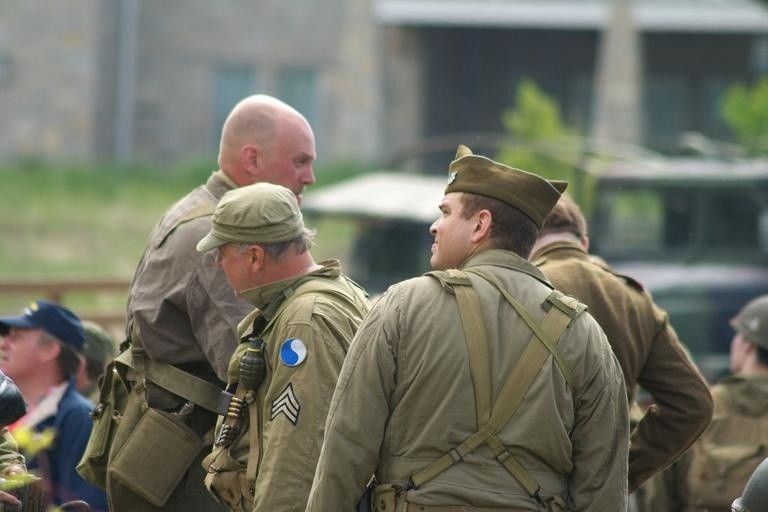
left=730, top=296, right=768, bottom=351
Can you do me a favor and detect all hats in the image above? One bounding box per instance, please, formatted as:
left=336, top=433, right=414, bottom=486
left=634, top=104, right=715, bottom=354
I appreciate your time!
left=196, top=183, right=304, bottom=251
left=445, top=144, right=568, bottom=230
left=85, top=320, right=116, bottom=360
left=0, top=300, right=86, bottom=349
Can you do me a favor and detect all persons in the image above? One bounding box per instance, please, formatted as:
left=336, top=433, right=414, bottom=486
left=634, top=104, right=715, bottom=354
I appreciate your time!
left=70, top=322, right=119, bottom=403
left=77, top=94, right=317, bottom=509
left=0, top=370, right=27, bottom=430
left=196, top=181, right=374, bottom=510
left=527, top=196, right=715, bottom=512
left=0, top=299, right=110, bottom=509
left=306, top=145, right=631, bottom=511
left=665, top=295, right=768, bottom=511
